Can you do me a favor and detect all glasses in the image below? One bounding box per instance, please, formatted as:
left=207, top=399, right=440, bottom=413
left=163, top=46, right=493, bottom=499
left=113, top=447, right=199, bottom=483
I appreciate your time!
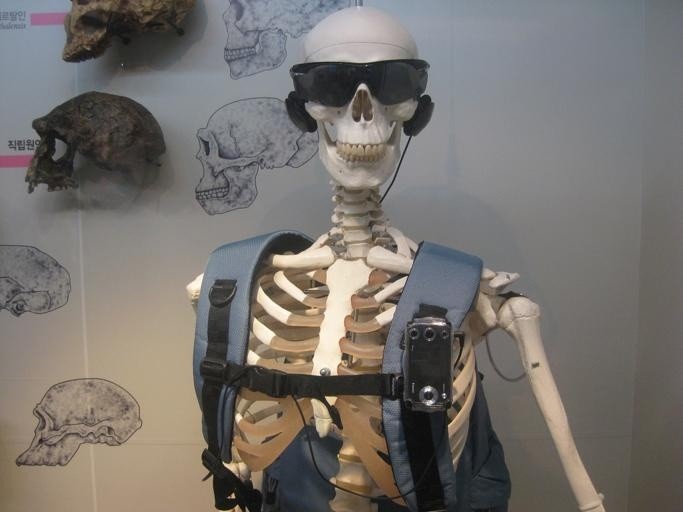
left=289, top=59, right=430, bottom=107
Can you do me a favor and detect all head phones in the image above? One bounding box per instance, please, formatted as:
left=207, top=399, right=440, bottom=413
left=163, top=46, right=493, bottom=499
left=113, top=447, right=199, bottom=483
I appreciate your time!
left=284, top=91, right=435, bottom=136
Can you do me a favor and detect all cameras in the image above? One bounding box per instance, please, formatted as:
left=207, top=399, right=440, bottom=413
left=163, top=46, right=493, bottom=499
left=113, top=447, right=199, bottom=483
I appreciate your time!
left=403, top=316, right=454, bottom=414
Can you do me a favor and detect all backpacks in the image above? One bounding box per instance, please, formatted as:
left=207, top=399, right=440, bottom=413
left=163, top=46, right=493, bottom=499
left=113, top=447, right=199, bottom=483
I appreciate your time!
left=192, top=227, right=512, bottom=512
